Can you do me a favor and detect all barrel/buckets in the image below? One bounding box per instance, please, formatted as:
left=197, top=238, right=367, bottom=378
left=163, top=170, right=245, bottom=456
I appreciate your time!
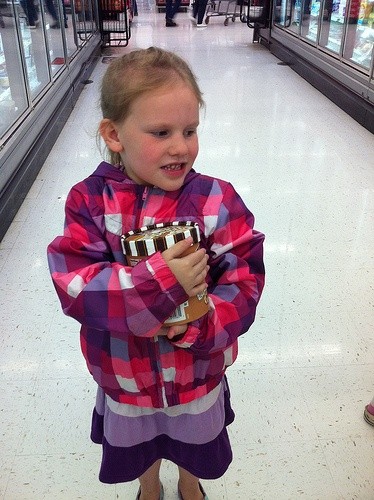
left=121, top=220, right=210, bottom=327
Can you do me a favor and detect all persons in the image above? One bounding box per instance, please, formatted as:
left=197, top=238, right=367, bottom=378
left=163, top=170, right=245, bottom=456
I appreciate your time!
left=192, top=0, right=209, bottom=27
left=44, top=0, right=68, bottom=29
left=165, top=0, right=181, bottom=27
left=18, top=0, right=39, bottom=29
left=46, top=47, right=265, bottom=500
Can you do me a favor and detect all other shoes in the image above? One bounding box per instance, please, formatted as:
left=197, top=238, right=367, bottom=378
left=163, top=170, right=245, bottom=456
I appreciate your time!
left=51, top=21, right=68, bottom=29
left=178, top=478, right=209, bottom=500
left=135, top=479, right=164, bottom=500
left=166, top=19, right=176, bottom=27
left=197, top=23, right=208, bottom=27
left=26, top=22, right=36, bottom=29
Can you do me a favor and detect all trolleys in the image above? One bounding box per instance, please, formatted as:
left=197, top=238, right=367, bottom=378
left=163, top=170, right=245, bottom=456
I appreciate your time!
left=204, top=0, right=238, bottom=27
